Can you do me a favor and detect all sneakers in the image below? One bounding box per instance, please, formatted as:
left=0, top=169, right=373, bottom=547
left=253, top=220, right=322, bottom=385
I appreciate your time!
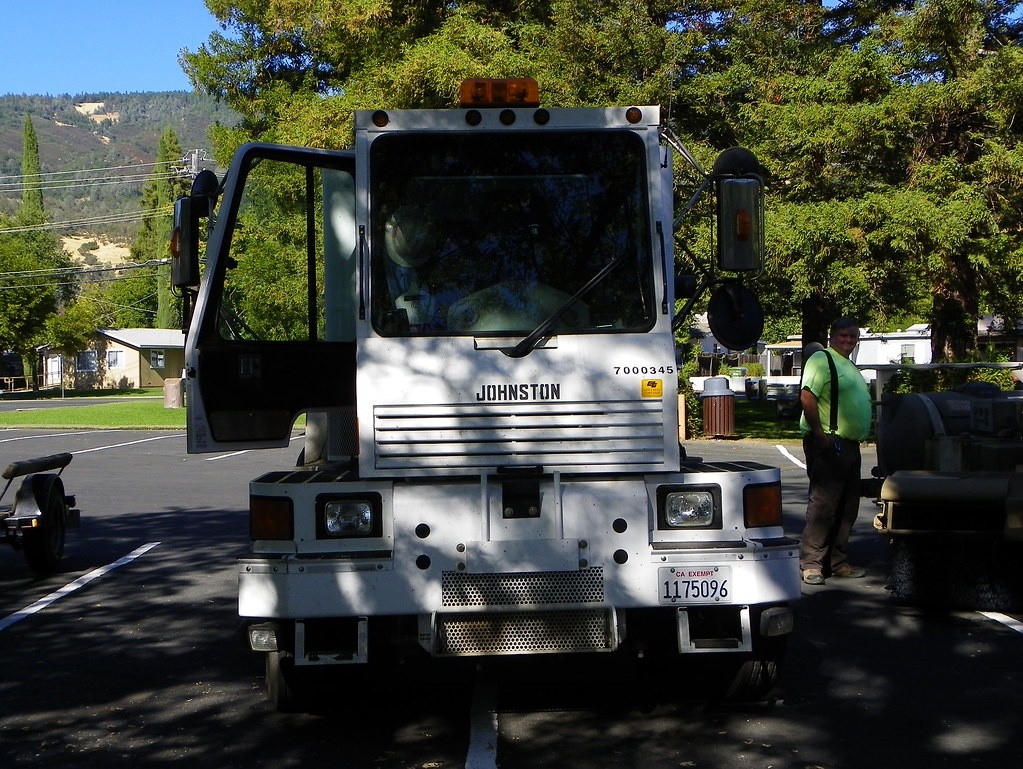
left=802, top=568, right=825, bottom=585
left=823, top=561, right=866, bottom=578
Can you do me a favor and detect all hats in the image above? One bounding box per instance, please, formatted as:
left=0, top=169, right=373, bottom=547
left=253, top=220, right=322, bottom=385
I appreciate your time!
left=383, top=204, right=440, bottom=267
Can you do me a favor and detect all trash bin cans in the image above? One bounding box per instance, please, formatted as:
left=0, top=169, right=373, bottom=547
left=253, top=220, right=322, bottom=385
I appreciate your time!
left=729, top=367, right=746, bottom=377
left=699, top=375, right=736, bottom=436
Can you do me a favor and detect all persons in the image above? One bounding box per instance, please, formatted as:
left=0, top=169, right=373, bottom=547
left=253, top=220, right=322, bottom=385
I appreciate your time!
left=799, top=315, right=872, bottom=583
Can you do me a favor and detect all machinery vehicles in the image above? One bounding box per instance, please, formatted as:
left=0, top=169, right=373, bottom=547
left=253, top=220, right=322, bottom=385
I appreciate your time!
left=169, top=77, right=806, bottom=715
